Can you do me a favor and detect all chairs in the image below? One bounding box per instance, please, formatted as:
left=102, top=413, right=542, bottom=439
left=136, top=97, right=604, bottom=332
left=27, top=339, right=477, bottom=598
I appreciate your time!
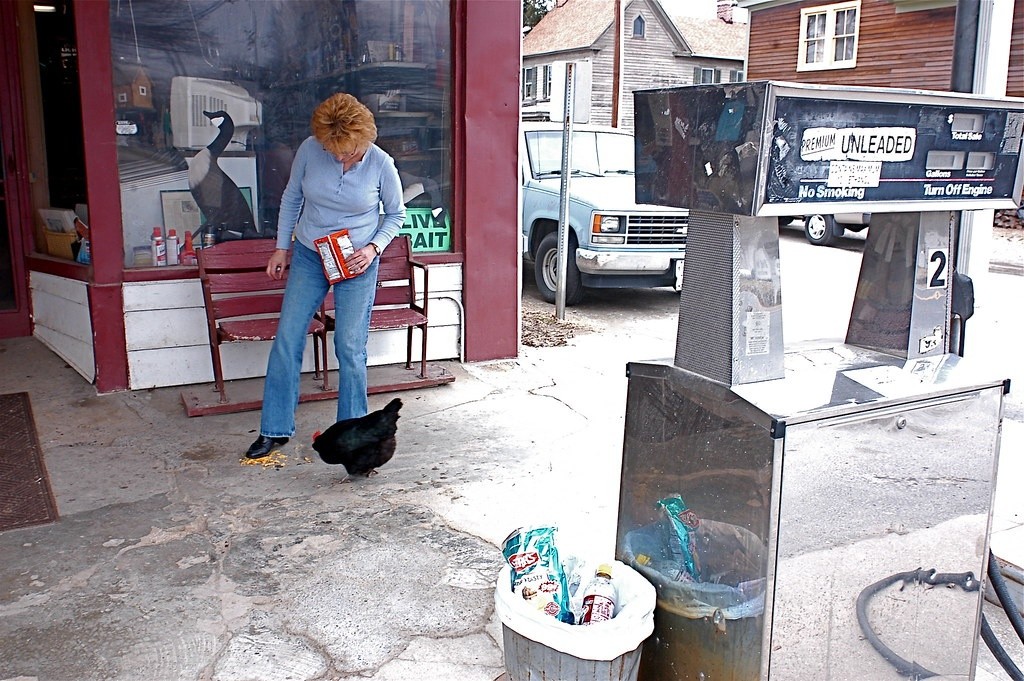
left=196, top=240, right=331, bottom=405
left=312, top=235, right=429, bottom=391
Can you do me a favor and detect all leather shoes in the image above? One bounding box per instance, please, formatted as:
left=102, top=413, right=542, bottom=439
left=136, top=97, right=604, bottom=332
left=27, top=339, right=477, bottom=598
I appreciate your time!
left=246, top=435, right=289, bottom=459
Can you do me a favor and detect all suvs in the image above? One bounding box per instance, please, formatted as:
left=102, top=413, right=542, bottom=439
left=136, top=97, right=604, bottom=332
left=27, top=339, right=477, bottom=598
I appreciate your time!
left=519, top=119, right=690, bottom=306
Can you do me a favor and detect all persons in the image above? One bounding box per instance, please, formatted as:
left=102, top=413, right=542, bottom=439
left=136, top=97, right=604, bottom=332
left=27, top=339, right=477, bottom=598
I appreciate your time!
left=246, top=93, right=407, bottom=459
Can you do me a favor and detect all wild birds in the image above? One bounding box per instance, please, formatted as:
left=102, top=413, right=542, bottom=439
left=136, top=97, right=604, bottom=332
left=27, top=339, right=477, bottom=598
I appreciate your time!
left=181, top=109, right=258, bottom=252
left=378, top=172, right=446, bottom=230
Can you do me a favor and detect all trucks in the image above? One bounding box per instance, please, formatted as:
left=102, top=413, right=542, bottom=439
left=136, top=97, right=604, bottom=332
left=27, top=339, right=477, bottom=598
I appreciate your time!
left=775, top=215, right=881, bottom=246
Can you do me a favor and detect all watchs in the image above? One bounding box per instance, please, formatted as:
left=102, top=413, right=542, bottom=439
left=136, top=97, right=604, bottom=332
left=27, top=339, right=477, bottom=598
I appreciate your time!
left=372, top=243, right=380, bottom=254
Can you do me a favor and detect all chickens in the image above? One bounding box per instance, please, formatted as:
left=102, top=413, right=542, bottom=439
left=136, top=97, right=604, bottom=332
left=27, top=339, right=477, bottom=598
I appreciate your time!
left=311, top=398, right=403, bottom=483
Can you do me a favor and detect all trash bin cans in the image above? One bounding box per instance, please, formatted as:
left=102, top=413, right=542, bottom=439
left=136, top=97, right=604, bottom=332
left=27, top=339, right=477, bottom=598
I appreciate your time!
left=625, top=520, right=767, bottom=680
left=497, top=559, right=655, bottom=681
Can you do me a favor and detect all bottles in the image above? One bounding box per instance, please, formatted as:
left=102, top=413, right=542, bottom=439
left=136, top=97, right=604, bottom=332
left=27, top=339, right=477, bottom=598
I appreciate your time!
left=133, top=209, right=273, bottom=267
left=637, top=555, right=700, bottom=587
left=360, top=42, right=403, bottom=65
left=581, top=563, right=616, bottom=625
